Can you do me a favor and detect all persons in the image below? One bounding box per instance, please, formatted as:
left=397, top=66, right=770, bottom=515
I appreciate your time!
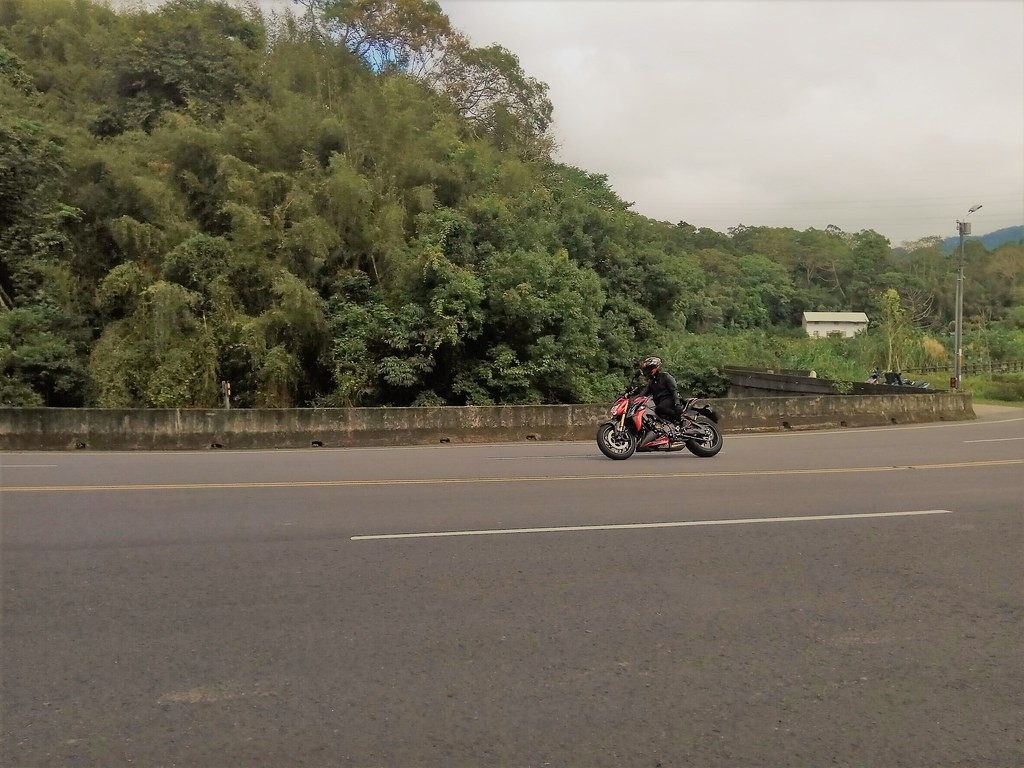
left=639, top=356, right=682, bottom=422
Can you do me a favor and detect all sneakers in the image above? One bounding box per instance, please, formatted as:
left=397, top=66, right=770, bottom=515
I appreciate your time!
left=673, top=418, right=684, bottom=427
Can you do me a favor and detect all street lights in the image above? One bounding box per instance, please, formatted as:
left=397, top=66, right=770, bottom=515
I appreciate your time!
left=956, top=205, right=982, bottom=390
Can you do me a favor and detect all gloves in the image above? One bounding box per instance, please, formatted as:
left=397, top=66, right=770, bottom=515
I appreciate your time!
left=674, top=404, right=685, bottom=416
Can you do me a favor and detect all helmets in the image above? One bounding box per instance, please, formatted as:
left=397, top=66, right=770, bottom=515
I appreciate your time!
left=641, top=356, right=663, bottom=380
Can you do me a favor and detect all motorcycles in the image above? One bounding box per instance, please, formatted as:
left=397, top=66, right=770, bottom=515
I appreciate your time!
left=867, top=367, right=931, bottom=388
left=596, top=369, right=723, bottom=460
